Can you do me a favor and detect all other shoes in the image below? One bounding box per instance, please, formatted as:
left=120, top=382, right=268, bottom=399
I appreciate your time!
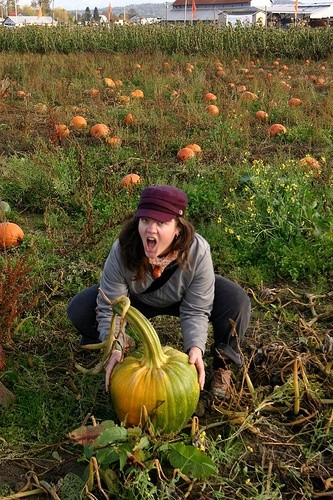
left=207, top=368, right=233, bottom=400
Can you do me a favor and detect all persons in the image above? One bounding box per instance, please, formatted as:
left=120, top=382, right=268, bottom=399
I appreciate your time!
left=68, top=187, right=251, bottom=400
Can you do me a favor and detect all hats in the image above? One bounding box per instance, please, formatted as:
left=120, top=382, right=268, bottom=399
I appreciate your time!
left=135, top=185, right=188, bottom=222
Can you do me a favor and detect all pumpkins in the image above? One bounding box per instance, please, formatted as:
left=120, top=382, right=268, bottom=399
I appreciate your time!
left=0, top=201, right=25, bottom=250
left=0, top=59, right=326, bottom=189
left=108, top=300, right=200, bottom=435
